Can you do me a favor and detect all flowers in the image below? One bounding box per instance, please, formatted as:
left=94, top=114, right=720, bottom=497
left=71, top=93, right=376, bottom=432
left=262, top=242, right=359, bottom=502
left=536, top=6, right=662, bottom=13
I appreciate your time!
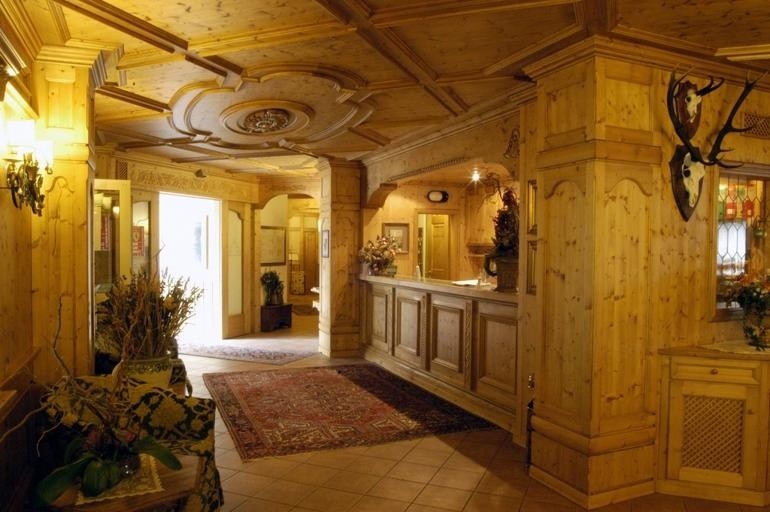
left=36, top=422, right=181, bottom=508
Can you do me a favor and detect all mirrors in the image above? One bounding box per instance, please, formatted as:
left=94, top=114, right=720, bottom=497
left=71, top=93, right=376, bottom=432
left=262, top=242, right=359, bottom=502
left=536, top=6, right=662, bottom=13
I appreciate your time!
left=132, top=200, right=152, bottom=282
left=92, top=185, right=121, bottom=293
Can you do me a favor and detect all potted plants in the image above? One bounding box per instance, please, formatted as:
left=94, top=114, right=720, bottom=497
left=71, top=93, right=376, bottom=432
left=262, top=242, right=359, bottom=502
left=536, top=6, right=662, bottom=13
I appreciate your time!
left=261, top=271, right=284, bottom=305
left=97, top=264, right=205, bottom=390
left=358, top=233, right=401, bottom=276
left=736, top=281, right=770, bottom=351
left=482, top=188, right=520, bottom=293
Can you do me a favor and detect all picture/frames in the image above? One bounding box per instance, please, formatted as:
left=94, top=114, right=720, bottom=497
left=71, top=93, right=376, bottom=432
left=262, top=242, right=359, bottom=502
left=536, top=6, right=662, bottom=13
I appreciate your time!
left=259, top=225, right=286, bottom=267
left=382, top=222, right=410, bottom=253
left=322, top=229, right=329, bottom=259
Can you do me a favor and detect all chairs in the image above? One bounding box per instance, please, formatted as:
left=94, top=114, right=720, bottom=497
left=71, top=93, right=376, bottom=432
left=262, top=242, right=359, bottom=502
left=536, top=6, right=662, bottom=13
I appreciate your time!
left=40, top=374, right=223, bottom=512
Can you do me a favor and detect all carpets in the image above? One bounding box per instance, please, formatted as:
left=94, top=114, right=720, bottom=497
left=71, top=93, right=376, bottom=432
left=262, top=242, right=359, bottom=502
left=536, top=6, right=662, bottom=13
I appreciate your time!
left=200, top=363, right=501, bottom=463
left=178, top=342, right=317, bottom=366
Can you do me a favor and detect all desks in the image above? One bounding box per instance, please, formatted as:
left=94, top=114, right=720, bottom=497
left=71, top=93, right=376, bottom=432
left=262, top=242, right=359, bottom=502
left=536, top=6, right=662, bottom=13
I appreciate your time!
left=41, top=446, right=210, bottom=512
left=260, top=303, right=293, bottom=332
